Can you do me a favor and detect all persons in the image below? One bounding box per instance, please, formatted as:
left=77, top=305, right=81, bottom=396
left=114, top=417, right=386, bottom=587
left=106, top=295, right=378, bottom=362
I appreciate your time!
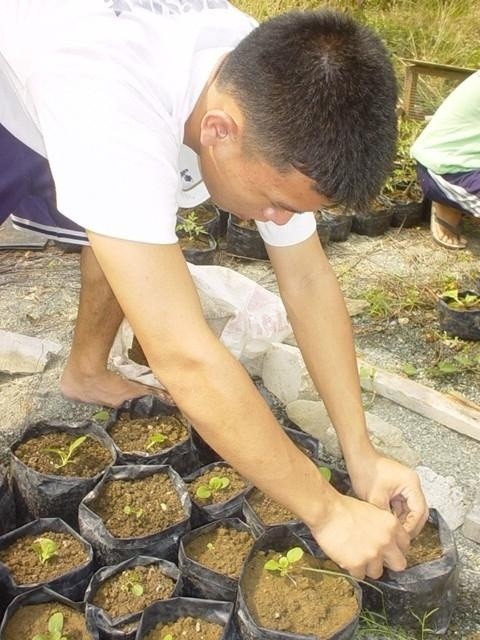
left=407, top=69, right=480, bottom=248
left=1, top=0, right=431, bottom=584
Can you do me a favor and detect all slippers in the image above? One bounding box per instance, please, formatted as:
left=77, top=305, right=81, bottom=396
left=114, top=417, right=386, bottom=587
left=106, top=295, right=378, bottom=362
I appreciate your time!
left=428, top=201, right=468, bottom=251
